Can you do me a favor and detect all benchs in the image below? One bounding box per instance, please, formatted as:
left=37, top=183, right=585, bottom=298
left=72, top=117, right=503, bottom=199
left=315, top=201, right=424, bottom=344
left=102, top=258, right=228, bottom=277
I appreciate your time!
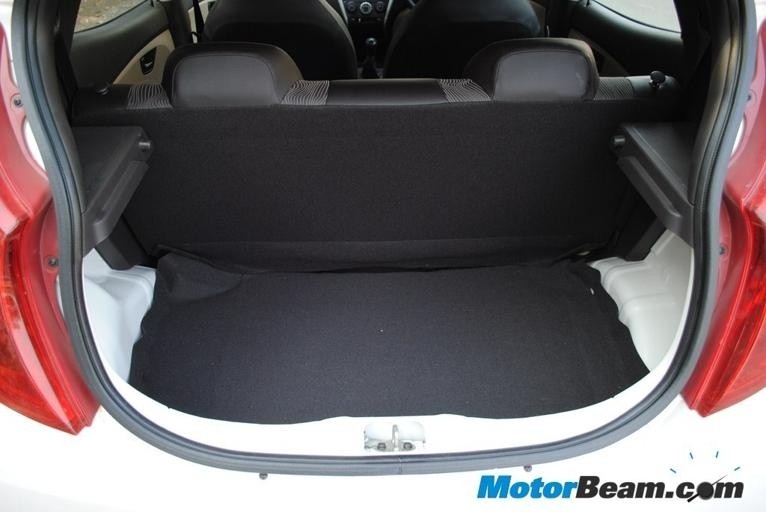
left=104, top=41, right=666, bottom=268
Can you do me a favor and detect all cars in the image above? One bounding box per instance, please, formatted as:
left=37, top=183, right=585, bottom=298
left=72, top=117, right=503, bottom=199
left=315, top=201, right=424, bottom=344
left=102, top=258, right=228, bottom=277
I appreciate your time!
left=0, top=0, right=766, bottom=500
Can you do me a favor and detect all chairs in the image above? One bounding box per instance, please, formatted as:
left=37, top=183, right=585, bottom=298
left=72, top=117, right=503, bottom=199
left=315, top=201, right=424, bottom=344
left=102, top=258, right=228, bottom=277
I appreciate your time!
left=383, top=0, right=546, bottom=79
left=204, top=1, right=359, bottom=79
